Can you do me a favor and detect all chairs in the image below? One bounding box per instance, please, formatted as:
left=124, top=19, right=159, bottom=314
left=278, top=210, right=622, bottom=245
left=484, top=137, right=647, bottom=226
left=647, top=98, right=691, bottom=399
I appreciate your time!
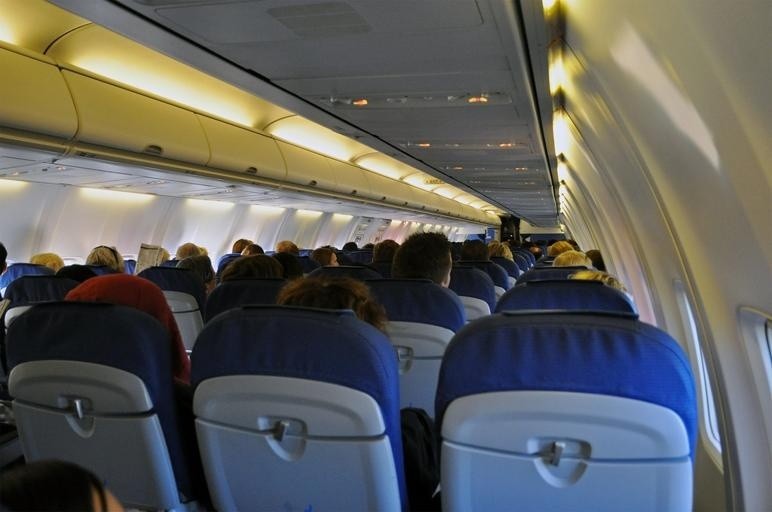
left=490, top=256, right=519, bottom=288
left=341, top=249, right=373, bottom=264
left=448, top=266, right=494, bottom=322
left=513, top=250, right=531, bottom=269
left=511, top=253, right=527, bottom=275
left=0, top=262, right=53, bottom=299
left=431, top=309, right=697, bottom=512
left=5, top=300, right=201, bottom=512
left=126, top=259, right=136, bottom=275
left=512, top=266, right=589, bottom=285
left=365, top=277, right=466, bottom=419
left=487, top=261, right=509, bottom=302
left=137, top=267, right=206, bottom=356
left=55, top=265, right=118, bottom=284
left=494, top=280, right=639, bottom=312
left=0, top=274, right=80, bottom=334
left=190, top=305, right=405, bottom=512
left=160, top=260, right=179, bottom=266
left=515, top=247, right=535, bottom=267
left=202, top=283, right=280, bottom=324
left=306, top=265, right=382, bottom=281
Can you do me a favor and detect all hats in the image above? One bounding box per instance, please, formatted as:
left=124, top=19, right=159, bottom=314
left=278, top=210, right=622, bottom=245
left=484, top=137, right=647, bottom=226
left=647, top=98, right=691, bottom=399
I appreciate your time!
left=60, top=273, right=191, bottom=380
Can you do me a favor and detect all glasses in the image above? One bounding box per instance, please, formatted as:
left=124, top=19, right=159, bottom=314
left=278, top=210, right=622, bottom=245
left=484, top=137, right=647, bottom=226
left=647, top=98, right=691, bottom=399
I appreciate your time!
left=95, top=246, right=120, bottom=263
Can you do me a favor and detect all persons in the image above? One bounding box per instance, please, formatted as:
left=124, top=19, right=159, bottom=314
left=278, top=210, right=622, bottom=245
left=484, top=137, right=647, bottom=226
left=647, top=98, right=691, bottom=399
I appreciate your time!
left=31, top=252, right=65, bottom=275
left=362, top=241, right=375, bottom=251
left=240, top=244, right=265, bottom=256
left=372, top=240, right=401, bottom=263
left=529, top=246, right=543, bottom=261
left=0, top=458, right=124, bottom=512
left=86, top=245, right=124, bottom=273
left=175, top=243, right=201, bottom=260
left=342, top=241, right=357, bottom=251
left=547, top=240, right=575, bottom=257
left=489, top=244, right=513, bottom=260
left=220, top=253, right=282, bottom=283
left=276, top=241, right=299, bottom=256
left=552, top=251, right=592, bottom=268
left=176, top=255, right=216, bottom=294
left=392, top=230, right=452, bottom=288
left=461, top=240, right=489, bottom=261
left=310, top=248, right=340, bottom=267
left=275, top=276, right=387, bottom=334
left=232, top=239, right=253, bottom=253
